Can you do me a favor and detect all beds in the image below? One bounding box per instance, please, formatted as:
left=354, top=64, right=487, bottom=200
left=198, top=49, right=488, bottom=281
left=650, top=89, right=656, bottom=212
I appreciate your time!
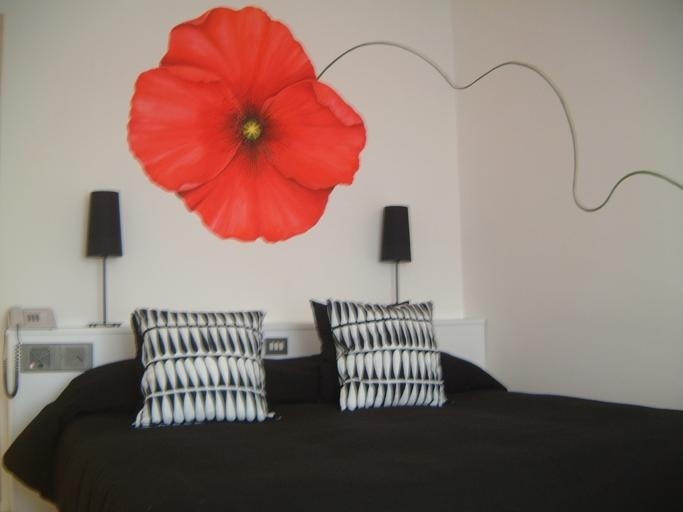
left=2, top=314, right=682, bottom=512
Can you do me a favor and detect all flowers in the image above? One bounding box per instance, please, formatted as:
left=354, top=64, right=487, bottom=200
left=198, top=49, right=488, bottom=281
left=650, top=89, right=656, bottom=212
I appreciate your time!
left=126, top=1, right=372, bottom=247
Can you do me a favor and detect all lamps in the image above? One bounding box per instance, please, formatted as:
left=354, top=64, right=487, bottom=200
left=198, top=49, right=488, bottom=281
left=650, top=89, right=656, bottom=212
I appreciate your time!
left=377, top=202, right=413, bottom=311
left=80, top=190, right=126, bottom=329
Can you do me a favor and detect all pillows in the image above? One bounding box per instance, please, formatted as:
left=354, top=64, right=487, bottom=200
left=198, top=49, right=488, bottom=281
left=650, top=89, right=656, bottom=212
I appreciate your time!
left=323, top=298, right=449, bottom=414
left=128, top=307, right=280, bottom=431
left=308, top=298, right=409, bottom=406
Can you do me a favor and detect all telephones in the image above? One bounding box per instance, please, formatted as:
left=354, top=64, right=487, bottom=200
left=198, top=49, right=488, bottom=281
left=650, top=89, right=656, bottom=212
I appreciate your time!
left=6, top=306, right=59, bottom=330
